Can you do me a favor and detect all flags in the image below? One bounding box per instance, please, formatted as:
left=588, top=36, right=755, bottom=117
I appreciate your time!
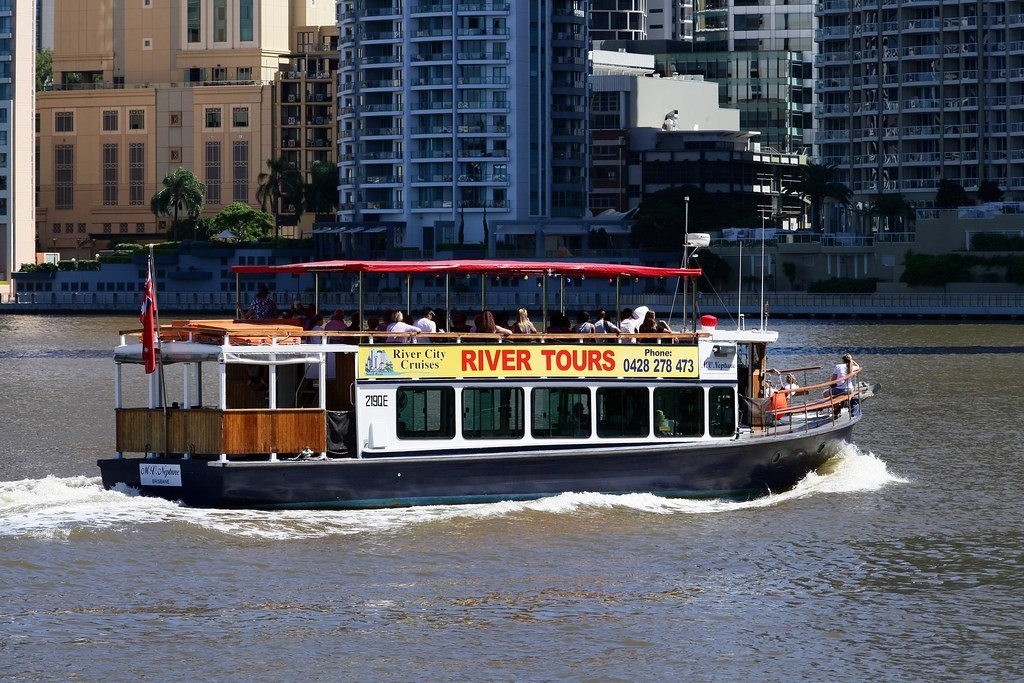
left=139, top=257, right=156, bottom=374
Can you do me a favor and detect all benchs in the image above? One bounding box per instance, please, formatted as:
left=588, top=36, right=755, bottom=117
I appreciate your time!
left=766, top=367, right=863, bottom=436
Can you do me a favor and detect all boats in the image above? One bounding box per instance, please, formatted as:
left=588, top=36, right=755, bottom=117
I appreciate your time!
left=95, top=260, right=878, bottom=513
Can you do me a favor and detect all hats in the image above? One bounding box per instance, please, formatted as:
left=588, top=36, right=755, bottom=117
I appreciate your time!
left=335, top=310, right=344, bottom=317
left=425, top=307, right=436, bottom=316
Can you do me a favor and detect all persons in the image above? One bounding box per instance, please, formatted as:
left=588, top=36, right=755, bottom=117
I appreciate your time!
left=547, top=311, right=571, bottom=332
left=574, top=311, right=595, bottom=341
left=595, top=309, right=621, bottom=343
left=784, top=374, right=799, bottom=396
left=386, top=310, right=421, bottom=343
left=772, top=384, right=786, bottom=419
left=620, top=308, right=644, bottom=343
left=655, top=410, right=668, bottom=430
left=830, top=353, right=860, bottom=420
left=568, top=402, right=588, bottom=424
left=639, top=311, right=679, bottom=343
left=511, top=308, right=537, bottom=333
left=283, top=293, right=388, bottom=344
left=413, top=308, right=436, bottom=343
left=236, top=286, right=278, bottom=320
left=431, top=308, right=513, bottom=334
left=765, top=380, right=774, bottom=397
left=657, top=320, right=676, bottom=343
